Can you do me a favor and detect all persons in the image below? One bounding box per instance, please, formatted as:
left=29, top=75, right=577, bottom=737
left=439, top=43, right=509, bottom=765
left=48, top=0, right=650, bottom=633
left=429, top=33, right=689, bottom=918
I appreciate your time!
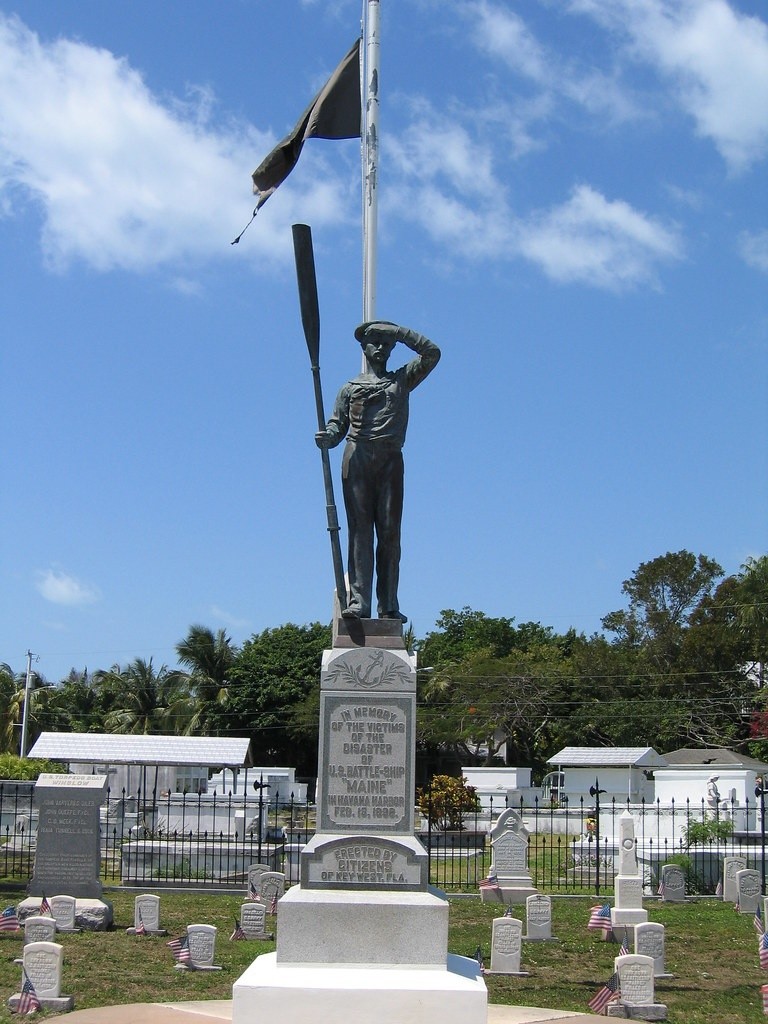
left=315, top=321, right=441, bottom=623
left=755, top=776, right=768, bottom=803
left=706, top=773, right=720, bottom=808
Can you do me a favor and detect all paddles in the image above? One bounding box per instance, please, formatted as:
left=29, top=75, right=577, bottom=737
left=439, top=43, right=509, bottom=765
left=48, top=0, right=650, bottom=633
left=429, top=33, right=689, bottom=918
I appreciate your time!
left=291, top=224, right=348, bottom=616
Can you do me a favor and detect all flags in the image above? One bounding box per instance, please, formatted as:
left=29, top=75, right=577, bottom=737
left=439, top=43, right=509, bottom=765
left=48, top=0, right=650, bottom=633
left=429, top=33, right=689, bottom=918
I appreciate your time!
left=715, top=879, right=722, bottom=895
left=473, top=947, right=483, bottom=970
left=166, top=937, right=191, bottom=963
left=759, top=932, right=768, bottom=970
left=503, top=907, right=512, bottom=917
left=618, top=936, right=629, bottom=955
left=588, top=904, right=613, bottom=931
left=762, top=985, right=768, bottom=1015
left=17, top=979, right=40, bottom=1015
left=479, top=876, right=498, bottom=893
left=40, top=898, right=49, bottom=916
left=0, top=906, right=20, bottom=930
left=587, top=972, right=618, bottom=1016
left=139, top=913, right=145, bottom=934
left=250, top=886, right=257, bottom=899
left=230, top=924, right=244, bottom=940
left=232, top=37, right=360, bottom=247
left=271, top=896, right=277, bottom=913
left=754, top=908, right=764, bottom=934
left=657, top=882, right=665, bottom=895
